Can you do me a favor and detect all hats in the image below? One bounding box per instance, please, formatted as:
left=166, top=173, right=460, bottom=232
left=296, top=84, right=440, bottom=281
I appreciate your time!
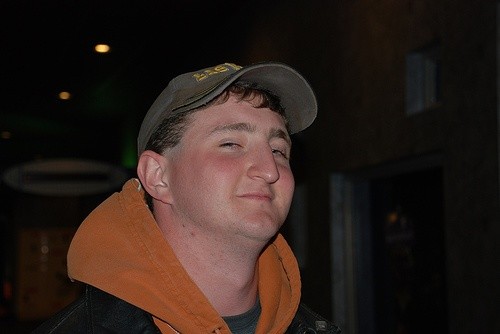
left=137, top=62, right=319, bottom=154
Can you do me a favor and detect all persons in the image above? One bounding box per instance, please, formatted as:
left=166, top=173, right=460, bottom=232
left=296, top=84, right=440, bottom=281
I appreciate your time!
left=46, top=62, right=348, bottom=334
